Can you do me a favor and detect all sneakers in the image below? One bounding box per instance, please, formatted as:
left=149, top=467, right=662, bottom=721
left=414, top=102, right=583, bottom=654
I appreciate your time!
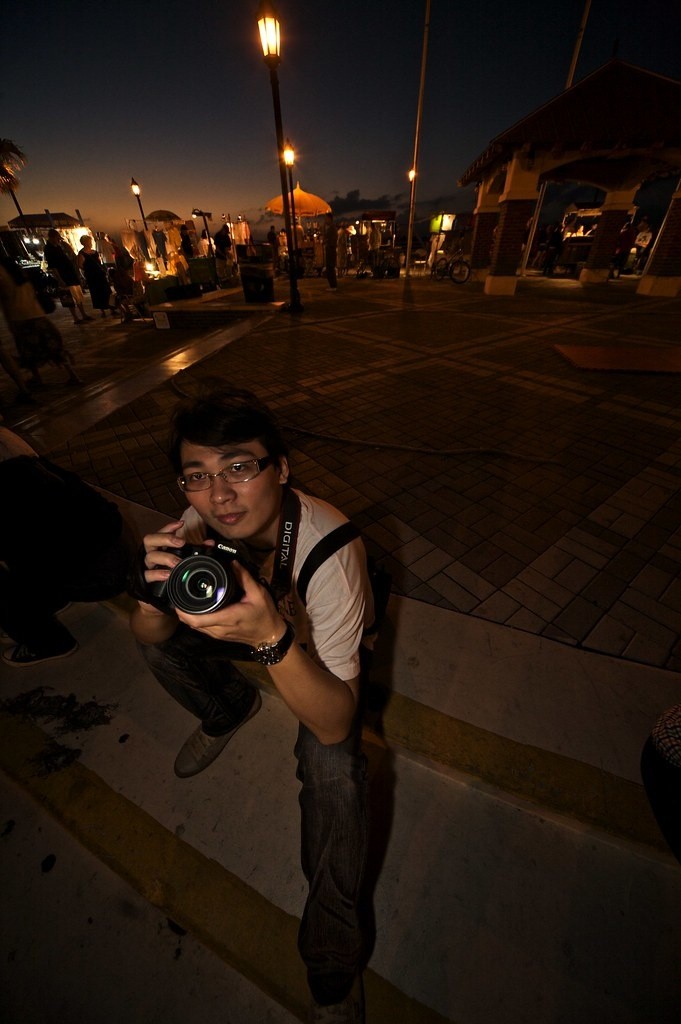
left=2, top=643, right=79, bottom=668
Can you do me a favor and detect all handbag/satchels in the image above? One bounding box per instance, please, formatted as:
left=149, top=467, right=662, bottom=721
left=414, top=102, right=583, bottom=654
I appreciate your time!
left=59, top=285, right=76, bottom=308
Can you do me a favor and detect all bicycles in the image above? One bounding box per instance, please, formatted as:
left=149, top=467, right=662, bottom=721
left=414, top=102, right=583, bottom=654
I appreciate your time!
left=434, top=228, right=471, bottom=284
left=356, top=244, right=400, bottom=279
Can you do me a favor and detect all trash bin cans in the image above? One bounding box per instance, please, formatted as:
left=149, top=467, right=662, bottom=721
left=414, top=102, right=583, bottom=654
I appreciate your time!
left=374, top=245, right=403, bottom=279
left=236, top=243, right=276, bottom=305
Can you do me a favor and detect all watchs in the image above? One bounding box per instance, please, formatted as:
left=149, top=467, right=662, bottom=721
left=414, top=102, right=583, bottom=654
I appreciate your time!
left=249, top=619, right=295, bottom=666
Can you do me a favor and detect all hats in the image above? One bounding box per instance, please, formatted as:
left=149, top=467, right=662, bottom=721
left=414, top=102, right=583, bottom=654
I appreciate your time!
left=346, top=226, right=356, bottom=235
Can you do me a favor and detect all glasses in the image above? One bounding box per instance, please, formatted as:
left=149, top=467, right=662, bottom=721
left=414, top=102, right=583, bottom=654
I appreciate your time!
left=176, top=453, right=273, bottom=492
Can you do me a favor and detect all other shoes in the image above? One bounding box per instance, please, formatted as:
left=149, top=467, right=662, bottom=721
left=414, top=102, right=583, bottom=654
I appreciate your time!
left=73, top=315, right=94, bottom=325
left=111, top=311, right=119, bottom=315
left=174, top=688, right=262, bottom=777
left=311, top=974, right=364, bottom=1024
left=101, top=313, right=105, bottom=318
left=326, top=288, right=336, bottom=291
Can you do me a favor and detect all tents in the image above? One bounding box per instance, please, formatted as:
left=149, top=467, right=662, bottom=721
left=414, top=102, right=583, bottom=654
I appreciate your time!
left=8, top=213, right=81, bottom=227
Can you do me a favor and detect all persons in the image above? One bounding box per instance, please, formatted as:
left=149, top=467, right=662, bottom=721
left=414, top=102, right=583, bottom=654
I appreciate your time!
left=320, top=213, right=390, bottom=291
left=0, top=229, right=150, bottom=389
left=515, top=214, right=652, bottom=279
left=0, top=427, right=138, bottom=665
left=267, top=216, right=304, bottom=279
left=180, top=224, right=231, bottom=279
left=131, top=389, right=380, bottom=1024
left=640, top=703, right=681, bottom=865
left=133, top=224, right=168, bottom=271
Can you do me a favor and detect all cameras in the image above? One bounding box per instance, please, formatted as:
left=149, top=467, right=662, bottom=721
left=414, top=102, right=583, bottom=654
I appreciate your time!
left=150, top=542, right=256, bottom=614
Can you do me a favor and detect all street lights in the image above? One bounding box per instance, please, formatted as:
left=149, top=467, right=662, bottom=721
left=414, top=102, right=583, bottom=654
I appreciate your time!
left=130, top=176, right=148, bottom=229
left=408, top=168, right=415, bottom=216
left=256, top=9, right=306, bottom=313
left=284, top=136, right=298, bottom=250
left=191, top=208, right=214, bottom=255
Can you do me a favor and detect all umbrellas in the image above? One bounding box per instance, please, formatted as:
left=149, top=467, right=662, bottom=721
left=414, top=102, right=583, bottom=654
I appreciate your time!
left=146, top=210, right=182, bottom=222
left=263, top=181, right=332, bottom=227
